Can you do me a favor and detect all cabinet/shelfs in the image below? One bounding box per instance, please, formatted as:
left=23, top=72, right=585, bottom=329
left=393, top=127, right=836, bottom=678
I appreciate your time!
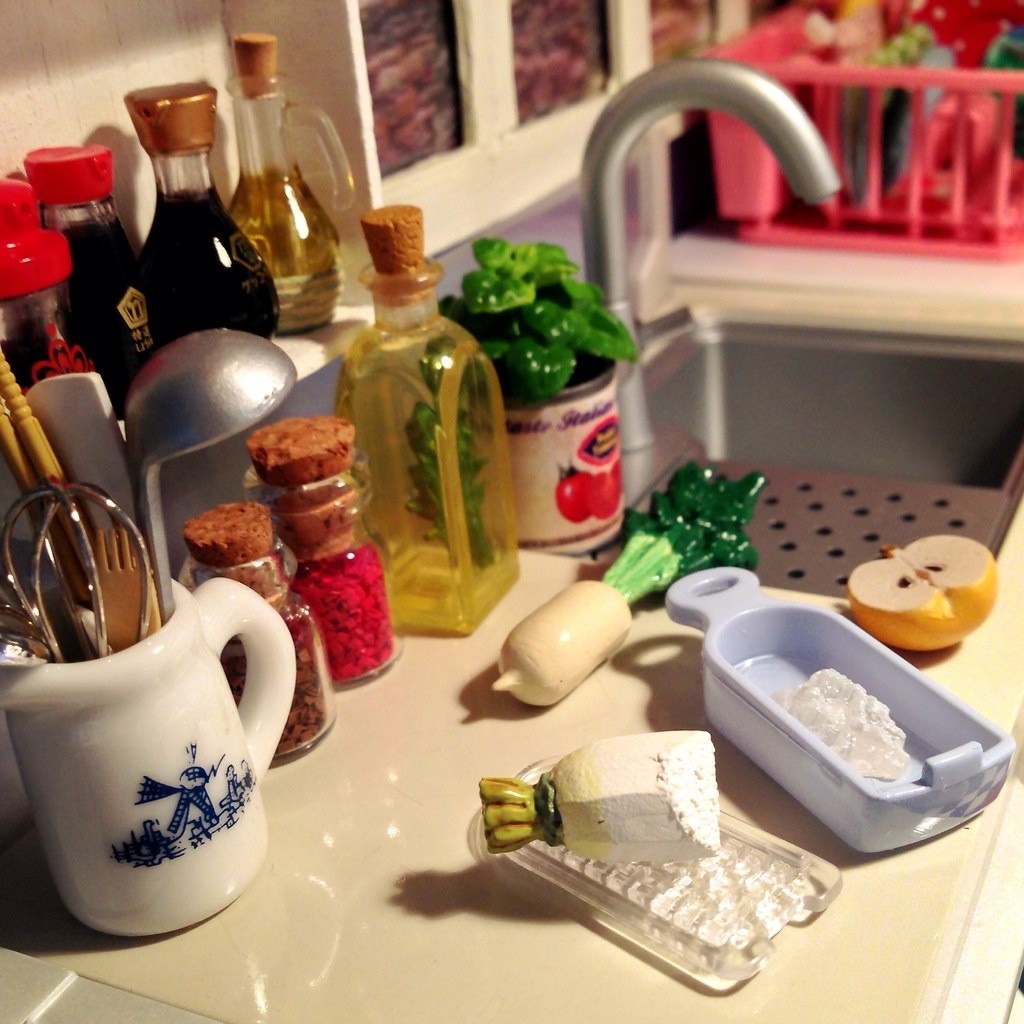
left=0, top=0, right=656, bottom=382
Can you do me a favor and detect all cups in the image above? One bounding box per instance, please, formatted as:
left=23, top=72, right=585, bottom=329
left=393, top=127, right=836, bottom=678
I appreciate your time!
left=0, top=578, right=297, bottom=935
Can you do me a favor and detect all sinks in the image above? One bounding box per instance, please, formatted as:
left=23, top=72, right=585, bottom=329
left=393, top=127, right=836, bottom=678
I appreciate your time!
left=617, top=303, right=1023, bottom=606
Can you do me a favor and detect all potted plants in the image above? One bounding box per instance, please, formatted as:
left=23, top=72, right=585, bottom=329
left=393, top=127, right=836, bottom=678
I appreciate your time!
left=401, top=236, right=625, bottom=567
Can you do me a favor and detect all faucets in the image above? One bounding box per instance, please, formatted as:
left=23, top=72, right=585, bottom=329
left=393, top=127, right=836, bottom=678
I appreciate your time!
left=577, top=60, right=844, bottom=451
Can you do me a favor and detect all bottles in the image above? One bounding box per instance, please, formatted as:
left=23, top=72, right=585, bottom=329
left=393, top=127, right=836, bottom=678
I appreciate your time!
left=23, top=146, right=177, bottom=419
left=178, top=500, right=338, bottom=756
left=338, top=205, right=521, bottom=638
left=125, top=85, right=282, bottom=349
left=241, top=419, right=400, bottom=686
left=0, top=177, right=93, bottom=392
left=225, top=32, right=356, bottom=339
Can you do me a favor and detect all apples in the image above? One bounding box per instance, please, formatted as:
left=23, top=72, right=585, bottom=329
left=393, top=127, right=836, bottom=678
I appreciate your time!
left=847, top=536, right=998, bottom=651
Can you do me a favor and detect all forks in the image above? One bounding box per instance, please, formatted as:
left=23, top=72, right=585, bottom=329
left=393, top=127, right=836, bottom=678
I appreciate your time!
left=96, top=528, right=161, bottom=653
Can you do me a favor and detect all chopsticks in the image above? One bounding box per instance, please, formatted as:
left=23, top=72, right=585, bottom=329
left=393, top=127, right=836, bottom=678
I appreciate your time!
left=0, top=347, right=93, bottom=604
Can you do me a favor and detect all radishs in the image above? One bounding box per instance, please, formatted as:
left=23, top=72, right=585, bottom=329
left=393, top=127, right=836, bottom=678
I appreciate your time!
left=491, top=465, right=756, bottom=706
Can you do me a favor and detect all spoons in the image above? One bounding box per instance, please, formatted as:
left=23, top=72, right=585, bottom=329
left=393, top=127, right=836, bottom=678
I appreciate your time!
left=128, top=330, right=297, bottom=626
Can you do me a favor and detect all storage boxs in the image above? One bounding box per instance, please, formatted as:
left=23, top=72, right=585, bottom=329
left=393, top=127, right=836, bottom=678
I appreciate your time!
left=705, top=0, right=1024, bottom=266
